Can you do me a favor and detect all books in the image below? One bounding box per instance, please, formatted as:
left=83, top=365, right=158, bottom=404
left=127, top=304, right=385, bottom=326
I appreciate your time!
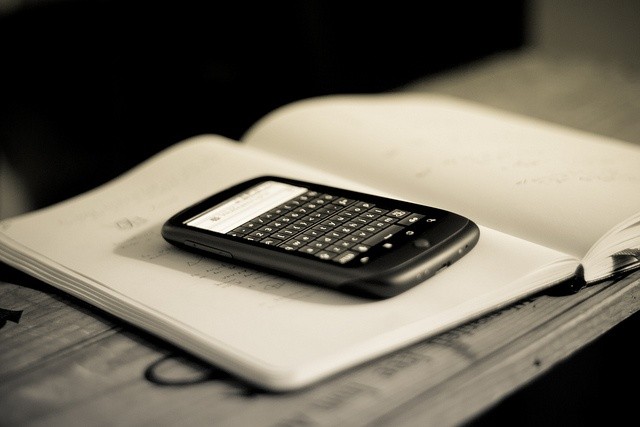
left=1, top=86, right=640, bottom=392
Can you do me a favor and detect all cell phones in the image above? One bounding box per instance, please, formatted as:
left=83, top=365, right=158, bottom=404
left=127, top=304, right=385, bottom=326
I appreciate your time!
left=161, top=173, right=481, bottom=300
